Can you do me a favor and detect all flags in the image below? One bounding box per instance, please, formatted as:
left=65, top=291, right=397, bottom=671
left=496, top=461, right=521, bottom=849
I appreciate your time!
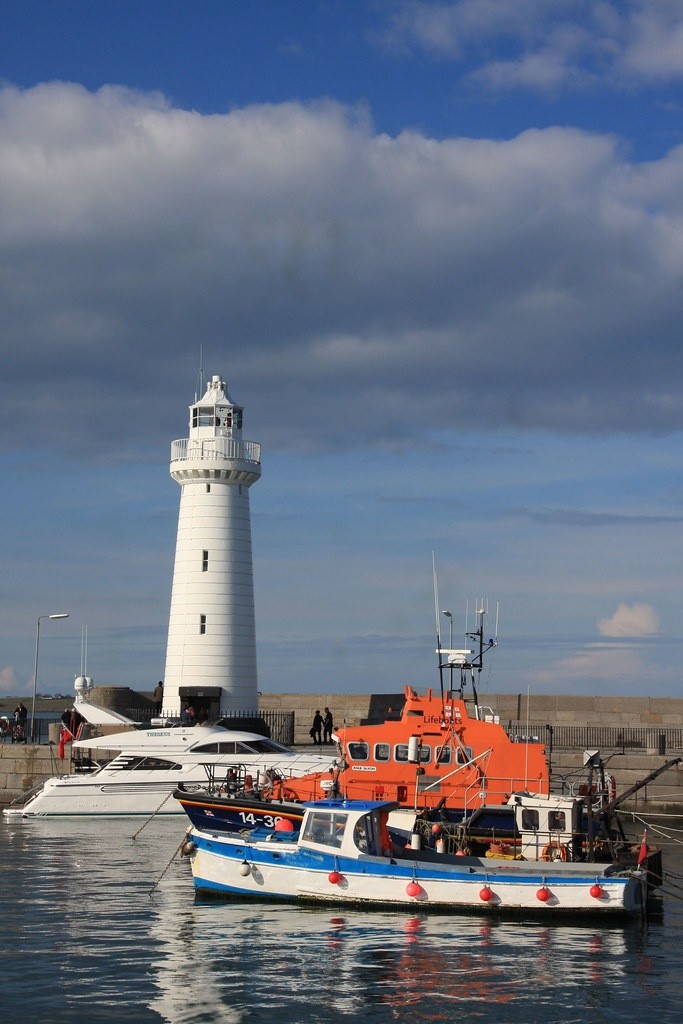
left=60, top=730, right=71, bottom=760
left=638, top=833, right=647, bottom=864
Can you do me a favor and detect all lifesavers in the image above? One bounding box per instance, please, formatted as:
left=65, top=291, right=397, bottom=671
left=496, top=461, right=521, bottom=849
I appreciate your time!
left=610, top=776, right=617, bottom=800
left=542, top=842, right=567, bottom=863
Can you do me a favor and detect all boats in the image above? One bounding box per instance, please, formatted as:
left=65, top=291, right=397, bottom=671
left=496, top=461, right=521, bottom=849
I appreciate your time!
left=173, top=724, right=656, bottom=923
left=4, top=723, right=338, bottom=820
left=180, top=544, right=669, bottom=889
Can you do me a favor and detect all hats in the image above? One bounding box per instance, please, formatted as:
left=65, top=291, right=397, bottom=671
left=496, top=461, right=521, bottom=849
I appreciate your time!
left=17, top=702, right=24, bottom=708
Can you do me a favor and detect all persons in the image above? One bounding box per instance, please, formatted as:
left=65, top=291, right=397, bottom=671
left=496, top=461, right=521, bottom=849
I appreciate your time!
left=61, top=708, right=81, bottom=738
left=154, top=681, right=163, bottom=715
left=182, top=704, right=196, bottom=724
left=14, top=702, right=27, bottom=728
left=323, top=707, right=333, bottom=743
left=311, top=710, right=325, bottom=743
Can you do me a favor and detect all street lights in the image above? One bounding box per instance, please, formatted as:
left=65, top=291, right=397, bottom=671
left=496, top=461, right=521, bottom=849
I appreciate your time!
left=31, top=613, right=71, bottom=741
left=442, top=609, right=454, bottom=691
